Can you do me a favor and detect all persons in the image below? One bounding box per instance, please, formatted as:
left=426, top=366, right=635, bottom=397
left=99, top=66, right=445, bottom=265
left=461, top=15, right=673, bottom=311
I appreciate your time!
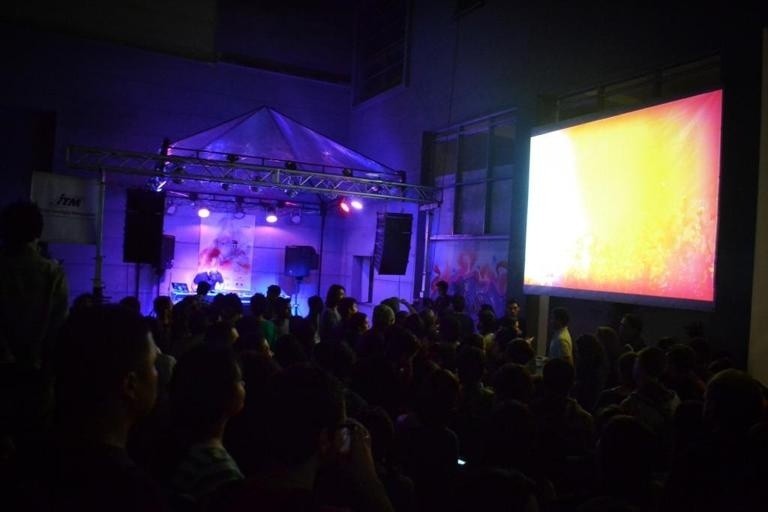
left=1, top=280, right=767, bottom=512
left=0, top=198, right=73, bottom=462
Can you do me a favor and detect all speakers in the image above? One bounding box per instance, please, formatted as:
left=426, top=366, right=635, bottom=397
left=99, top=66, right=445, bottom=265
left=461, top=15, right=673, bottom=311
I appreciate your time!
left=371, top=210, right=414, bottom=275
left=121, top=191, right=176, bottom=266
left=284, top=244, right=319, bottom=277
